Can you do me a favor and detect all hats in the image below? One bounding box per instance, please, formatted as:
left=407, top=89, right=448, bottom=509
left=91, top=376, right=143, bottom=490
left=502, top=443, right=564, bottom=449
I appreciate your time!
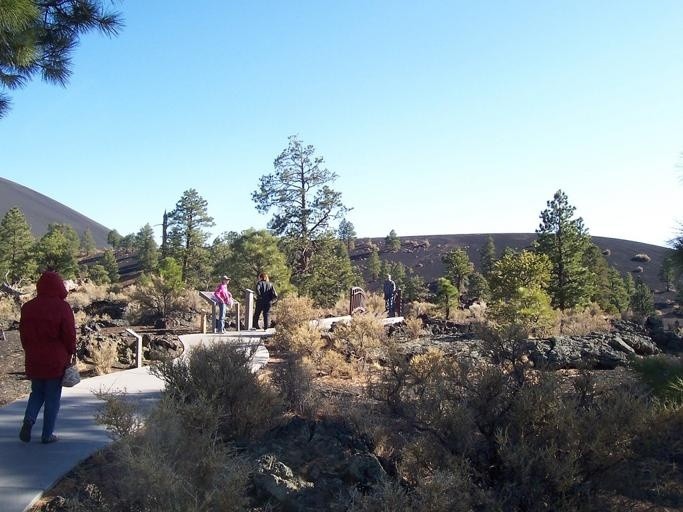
left=222, top=276, right=231, bottom=280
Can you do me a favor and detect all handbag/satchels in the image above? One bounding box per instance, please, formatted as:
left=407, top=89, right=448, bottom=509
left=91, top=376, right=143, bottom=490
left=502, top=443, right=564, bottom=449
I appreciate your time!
left=62, top=365, right=81, bottom=386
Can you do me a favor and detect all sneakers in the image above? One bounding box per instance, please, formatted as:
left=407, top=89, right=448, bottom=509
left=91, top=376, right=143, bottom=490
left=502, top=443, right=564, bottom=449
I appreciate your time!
left=41, top=433, right=57, bottom=442
left=20, top=419, right=34, bottom=443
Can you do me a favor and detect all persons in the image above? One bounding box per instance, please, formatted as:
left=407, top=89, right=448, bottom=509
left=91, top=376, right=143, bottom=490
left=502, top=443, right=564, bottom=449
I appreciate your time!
left=214, top=275, right=232, bottom=333
left=253, top=272, right=277, bottom=330
left=384, top=274, right=396, bottom=317
left=19, top=271, right=76, bottom=441
